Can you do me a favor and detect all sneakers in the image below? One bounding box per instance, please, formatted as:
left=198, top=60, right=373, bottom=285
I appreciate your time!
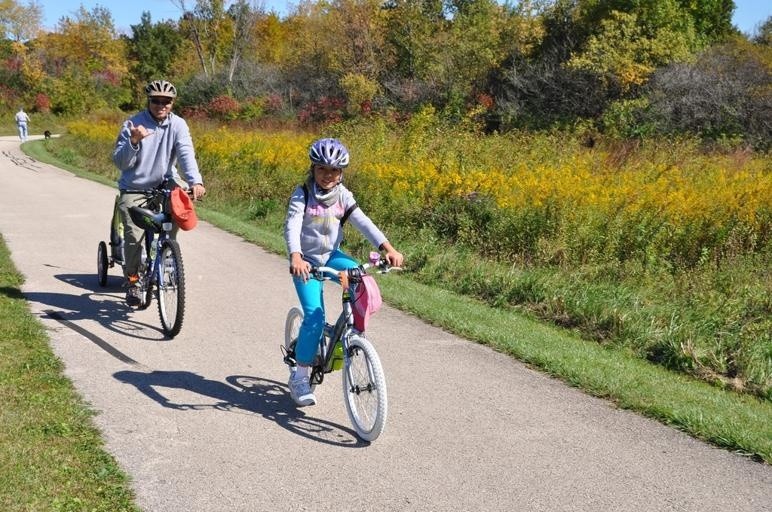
left=126, top=286, right=141, bottom=306
left=288, top=371, right=316, bottom=407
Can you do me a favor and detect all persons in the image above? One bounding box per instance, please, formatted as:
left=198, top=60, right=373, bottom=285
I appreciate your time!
left=285, top=138, right=404, bottom=404
left=15, top=108, right=31, bottom=142
left=113, top=80, right=206, bottom=308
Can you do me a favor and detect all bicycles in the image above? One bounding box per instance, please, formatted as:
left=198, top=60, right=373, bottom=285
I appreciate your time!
left=97, top=179, right=206, bottom=337
left=279, top=258, right=406, bottom=442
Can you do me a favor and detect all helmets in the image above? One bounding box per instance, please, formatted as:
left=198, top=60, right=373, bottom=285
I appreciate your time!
left=145, top=80, right=177, bottom=98
left=128, top=206, right=171, bottom=233
left=309, top=138, right=350, bottom=170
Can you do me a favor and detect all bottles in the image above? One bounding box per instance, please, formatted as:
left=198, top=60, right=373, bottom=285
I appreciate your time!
left=329, top=342, right=344, bottom=370
left=150, top=239, right=158, bottom=260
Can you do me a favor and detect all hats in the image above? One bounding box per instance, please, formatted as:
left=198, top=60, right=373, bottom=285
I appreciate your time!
left=351, top=275, right=382, bottom=331
left=168, top=187, right=198, bottom=230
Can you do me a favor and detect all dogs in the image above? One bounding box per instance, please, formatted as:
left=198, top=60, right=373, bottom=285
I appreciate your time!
left=44, top=130, right=52, bottom=140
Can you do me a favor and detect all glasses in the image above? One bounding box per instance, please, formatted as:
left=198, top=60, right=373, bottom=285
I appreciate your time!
left=149, top=97, right=172, bottom=105
